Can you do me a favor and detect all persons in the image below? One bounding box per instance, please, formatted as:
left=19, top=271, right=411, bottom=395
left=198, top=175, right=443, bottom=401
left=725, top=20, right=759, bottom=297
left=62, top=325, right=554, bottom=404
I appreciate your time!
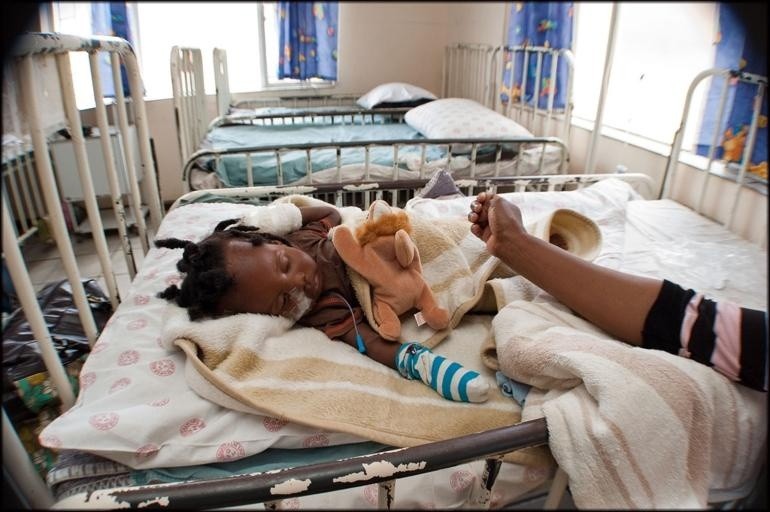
left=469, top=192, right=766, bottom=394
left=177, top=208, right=567, bottom=402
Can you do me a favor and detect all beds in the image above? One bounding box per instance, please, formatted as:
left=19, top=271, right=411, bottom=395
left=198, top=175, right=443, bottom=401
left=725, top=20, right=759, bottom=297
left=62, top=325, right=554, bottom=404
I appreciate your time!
left=173, top=46, right=575, bottom=192
left=2, top=34, right=768, bottom=510
left=206, top=46, right=498, bottom=115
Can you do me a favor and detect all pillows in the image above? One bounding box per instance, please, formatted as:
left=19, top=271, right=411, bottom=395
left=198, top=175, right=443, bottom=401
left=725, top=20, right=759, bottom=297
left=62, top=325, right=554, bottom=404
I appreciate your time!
left=356, top=82, right=439, bottom=109
left=404, top=98, right=536, bottom=153
left=38, top=204, right=371, bottom=470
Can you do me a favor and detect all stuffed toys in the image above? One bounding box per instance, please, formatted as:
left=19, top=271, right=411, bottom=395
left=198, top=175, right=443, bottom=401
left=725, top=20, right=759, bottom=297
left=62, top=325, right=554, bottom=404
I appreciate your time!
left=332, top=201, right=450, bottom=338
